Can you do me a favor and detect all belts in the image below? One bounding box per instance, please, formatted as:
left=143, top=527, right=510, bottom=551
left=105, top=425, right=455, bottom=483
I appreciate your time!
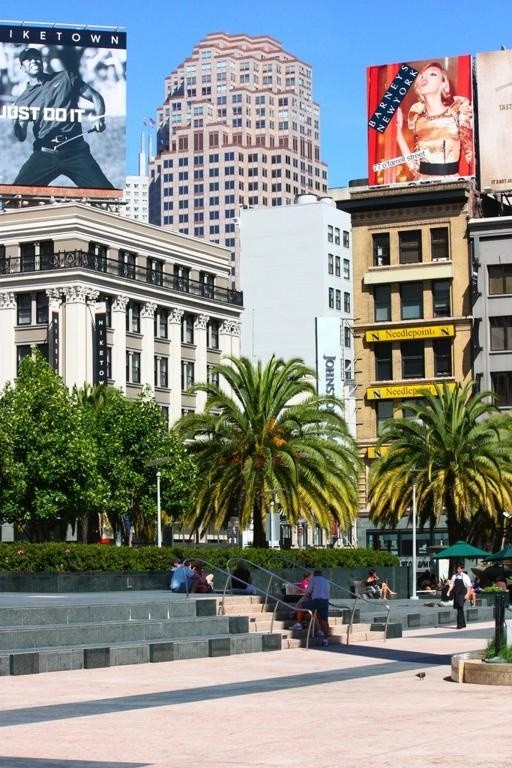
left=49, top=135, right=71, bottom=144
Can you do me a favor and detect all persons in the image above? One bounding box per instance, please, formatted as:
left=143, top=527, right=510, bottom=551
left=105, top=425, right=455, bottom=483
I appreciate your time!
left=10, top=48, right=114, bottom=190
left=170, top=558, right=216, bottom=593
left=496, top=577, right=507, bottom=590
left=471, top=574, right=480, bottom=607
left=439, top=580, right=455, bottom=607
left=366, top=569, right=397, bottom=600
left=396, top=62, right=473, bottom=182
left=288, top=570, right=331, bottom=647
left=296, top=572, right=312, bottom=593
left=446, top=562, right=472, bottom=629
left=230, top=560, right=257, bottom=594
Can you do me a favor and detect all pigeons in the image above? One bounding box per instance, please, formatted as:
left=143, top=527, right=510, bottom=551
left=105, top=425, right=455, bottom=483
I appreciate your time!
left=415, top=672, right=425, bottom=681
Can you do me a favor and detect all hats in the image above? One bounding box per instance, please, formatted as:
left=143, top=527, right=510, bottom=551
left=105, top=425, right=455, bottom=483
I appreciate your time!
left=20, top=48, right=41, bottom=62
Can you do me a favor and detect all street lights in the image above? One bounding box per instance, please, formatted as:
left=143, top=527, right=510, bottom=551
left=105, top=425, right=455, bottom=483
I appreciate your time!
left=402, top=468, right=428, bottom=599
left=144, top=457, right=171, bottom=547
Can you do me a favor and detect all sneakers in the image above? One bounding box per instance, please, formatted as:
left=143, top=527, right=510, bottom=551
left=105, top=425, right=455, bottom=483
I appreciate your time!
left=289, top=623, right=303, bottom=631
left=322, top=639, right=329, bottom=647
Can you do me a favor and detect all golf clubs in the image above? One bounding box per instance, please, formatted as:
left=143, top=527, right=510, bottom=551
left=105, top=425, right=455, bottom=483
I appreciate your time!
left=54, top=128, right=97, bottom=160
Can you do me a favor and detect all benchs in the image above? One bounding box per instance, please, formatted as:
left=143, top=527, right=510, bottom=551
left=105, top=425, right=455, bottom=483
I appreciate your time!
left=351, top=579, right=379, bottom=597
left=282, top=583, right=308, bottom=602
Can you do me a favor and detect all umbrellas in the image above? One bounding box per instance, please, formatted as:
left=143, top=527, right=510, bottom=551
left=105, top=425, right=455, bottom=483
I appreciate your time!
left=431, top=540, right=492, bottom=559
left=481, top=544, right=512, bottom=562
